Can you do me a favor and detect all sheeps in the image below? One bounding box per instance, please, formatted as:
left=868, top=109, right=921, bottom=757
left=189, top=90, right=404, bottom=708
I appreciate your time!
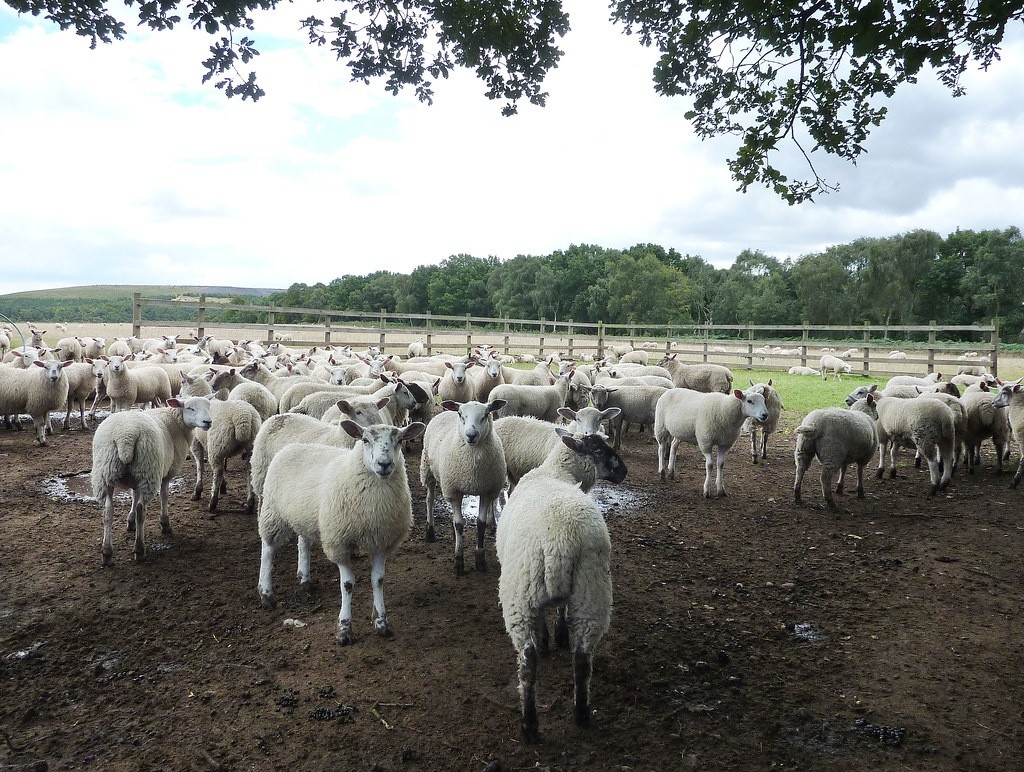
left=0, top=321, right=107, bottom=367
left=843, top=373, right=968, bottom=495
left=746, top=379, right=781, bottom=464
left=108, top=323, right=302, bottom=419
left=556, top=340, right=734, bottom=448
left=992, top=377, right=1024, bottom=489
left=958, top=381, right=1008, bottom=474
left=495, top=429, right=628, bottom=732
left=952, top=352, right=994, bottom=388
left=889, top=351, right=906, bottom=361
left=737, top=345, right=858, bottom=383
left=402, top=337, right=621, bottom=495
left=62, top=358, right=107, bottom=431
left=654, top=387, right=769, bottom=498
left=0, top=359, right=74, bottom=446
left=420, top=399, right=508, bottom=574
left=793, top=393, right=879, bottom=509
left=258, top=419, right=425, bottom=646
left=90, top=390, right=221, bottom=567
left=180, top=346, right=405, bottom=512
